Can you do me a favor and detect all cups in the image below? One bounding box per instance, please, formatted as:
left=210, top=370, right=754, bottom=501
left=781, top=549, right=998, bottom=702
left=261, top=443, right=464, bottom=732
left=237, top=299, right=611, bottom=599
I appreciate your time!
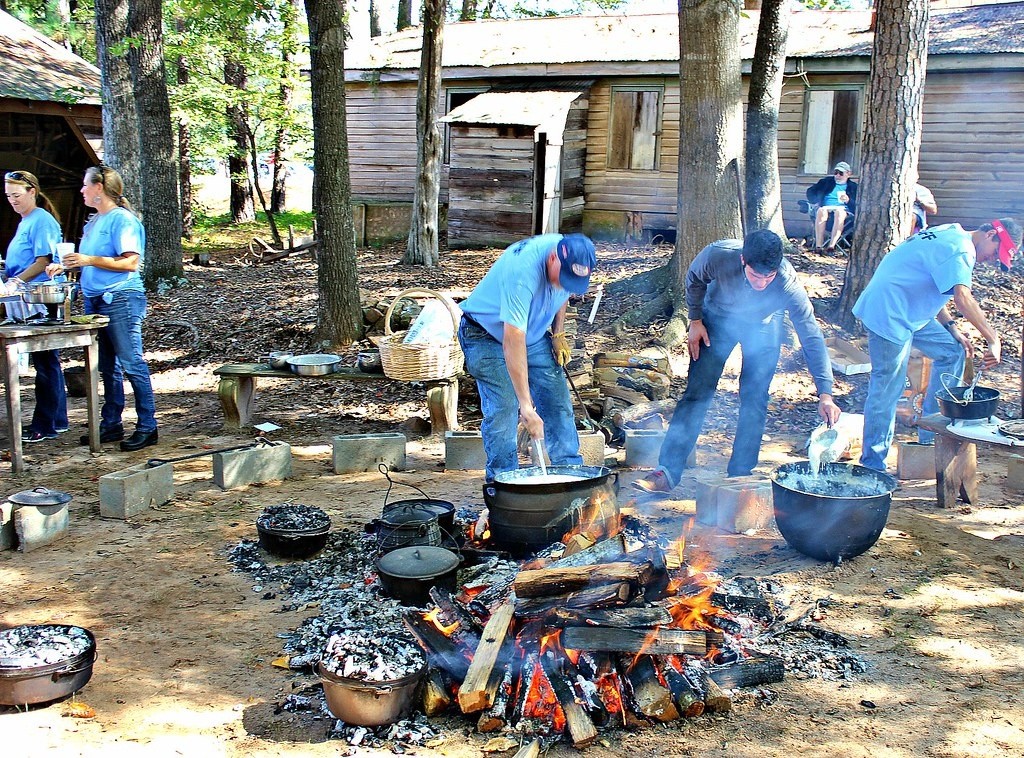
left=56, top=243, right=75, bottom=270
left=837, top=191, right=846, bottom=204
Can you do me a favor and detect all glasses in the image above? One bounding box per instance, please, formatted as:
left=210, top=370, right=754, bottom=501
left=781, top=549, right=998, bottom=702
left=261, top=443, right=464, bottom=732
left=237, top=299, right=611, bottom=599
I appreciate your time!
left=95, top=164, right=106, bottom=190
left=4, top=172, right=35, bottom=187
left=833, top=170, right=844, bottom=176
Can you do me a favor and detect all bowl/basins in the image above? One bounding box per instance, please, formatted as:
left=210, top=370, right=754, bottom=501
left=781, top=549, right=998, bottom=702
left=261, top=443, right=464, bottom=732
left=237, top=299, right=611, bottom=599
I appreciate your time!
left=358, top=349, right=382, bottom=373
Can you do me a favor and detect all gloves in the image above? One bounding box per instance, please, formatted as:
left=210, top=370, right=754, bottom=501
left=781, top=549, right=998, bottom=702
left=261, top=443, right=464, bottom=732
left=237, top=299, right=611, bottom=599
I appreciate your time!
left=548, top=330, right=571, bottom=367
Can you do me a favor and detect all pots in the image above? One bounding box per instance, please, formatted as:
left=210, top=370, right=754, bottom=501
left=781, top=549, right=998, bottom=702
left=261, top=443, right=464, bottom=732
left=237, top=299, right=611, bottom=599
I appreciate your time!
left=933, top=372, right=1002, bottom=427
left=770, top=460, right=898, bottom=561
left=311, top=638, right=429, bottom=727
left=65, top=366, right=88, bottom=397
left=482, top=465, right=618, bottom=558
left=0, top=625, right=99, bottom=705
left=375, top=519, right=464, bottom=607
left=376, top=506, right=442, bottom=555
left=255, top=512, right=331, bottom=560
left=377, top=463, right=455, bottom=540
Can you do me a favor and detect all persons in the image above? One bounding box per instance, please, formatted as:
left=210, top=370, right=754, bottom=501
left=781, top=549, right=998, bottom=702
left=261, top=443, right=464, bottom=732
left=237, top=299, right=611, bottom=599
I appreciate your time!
left=457, top=232, right=596, bottom=484
left=45, top=166, right=158, bottom=451
left=632, top=227, right=841, bottom=499
left=1, top=170, right=69, bottom=443
left=909, top=173, right=938, bottom=236
left=805, top=161, right=858, bottom=257
left=851, top=217, right=1022, bottom=493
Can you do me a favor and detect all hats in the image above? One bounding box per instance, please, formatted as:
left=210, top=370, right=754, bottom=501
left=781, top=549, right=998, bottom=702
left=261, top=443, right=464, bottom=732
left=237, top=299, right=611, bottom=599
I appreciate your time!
left=557, top=233, right=596, bottom=294
left=834, top=161, right=851, bottom=173
left=991, top=219, right=1017, bottom=268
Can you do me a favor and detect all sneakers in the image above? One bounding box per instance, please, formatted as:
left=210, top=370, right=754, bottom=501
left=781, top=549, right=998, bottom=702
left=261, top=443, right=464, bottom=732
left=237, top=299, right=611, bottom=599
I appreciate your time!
left=21, top=425, right=68, bottom=442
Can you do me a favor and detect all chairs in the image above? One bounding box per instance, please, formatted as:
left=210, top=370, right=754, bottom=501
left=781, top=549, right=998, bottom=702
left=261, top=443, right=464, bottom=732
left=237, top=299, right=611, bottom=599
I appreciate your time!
left=798, top=200, right=854, bottom=258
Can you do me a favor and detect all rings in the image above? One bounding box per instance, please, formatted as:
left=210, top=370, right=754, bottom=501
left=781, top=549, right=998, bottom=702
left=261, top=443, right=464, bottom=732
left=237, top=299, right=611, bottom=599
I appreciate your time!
left=73, top=260, right=78, bottom=264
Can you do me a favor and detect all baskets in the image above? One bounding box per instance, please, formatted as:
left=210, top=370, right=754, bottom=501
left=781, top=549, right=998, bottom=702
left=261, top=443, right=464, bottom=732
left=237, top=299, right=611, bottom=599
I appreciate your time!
left=369, top=288, right=465, bottom=383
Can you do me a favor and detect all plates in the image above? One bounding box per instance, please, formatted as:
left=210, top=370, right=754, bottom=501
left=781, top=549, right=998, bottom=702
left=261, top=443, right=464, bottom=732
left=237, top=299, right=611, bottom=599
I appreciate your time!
left=286, top=354, right=343, bottom=376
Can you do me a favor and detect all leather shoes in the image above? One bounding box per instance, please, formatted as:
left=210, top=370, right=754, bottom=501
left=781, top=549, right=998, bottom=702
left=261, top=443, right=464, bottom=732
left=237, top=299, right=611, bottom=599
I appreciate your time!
left=120, top=427, right=158, bottom=450
left=80, top=423, right=125, bottom=445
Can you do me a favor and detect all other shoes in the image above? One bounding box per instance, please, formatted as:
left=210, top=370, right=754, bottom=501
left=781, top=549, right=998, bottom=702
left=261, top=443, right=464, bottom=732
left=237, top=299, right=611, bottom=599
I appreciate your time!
left=815, top=247, right=835, bottom=255
left=630, top=470, right=672, bottom=495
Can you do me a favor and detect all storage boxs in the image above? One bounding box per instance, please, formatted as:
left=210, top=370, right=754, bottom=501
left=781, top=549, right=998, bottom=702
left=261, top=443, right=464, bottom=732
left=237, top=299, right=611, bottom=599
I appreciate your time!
left=822, top=335, right=871, bottom=373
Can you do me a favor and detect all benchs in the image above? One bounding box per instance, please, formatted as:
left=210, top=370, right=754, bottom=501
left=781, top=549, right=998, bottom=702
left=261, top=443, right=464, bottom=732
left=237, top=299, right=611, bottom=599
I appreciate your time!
left=212, top=362, right=466, bottom=437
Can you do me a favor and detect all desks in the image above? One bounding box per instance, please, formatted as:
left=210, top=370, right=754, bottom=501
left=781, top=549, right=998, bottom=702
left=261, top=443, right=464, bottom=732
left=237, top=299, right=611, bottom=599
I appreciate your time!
left=0, top=321, right=109, bottom=475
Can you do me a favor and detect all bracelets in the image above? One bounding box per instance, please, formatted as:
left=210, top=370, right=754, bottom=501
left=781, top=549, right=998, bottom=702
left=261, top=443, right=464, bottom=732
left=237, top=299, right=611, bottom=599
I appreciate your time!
left=944, top=320, right=955, bottom=329
left=919, top=201, right=924, bottom=207
